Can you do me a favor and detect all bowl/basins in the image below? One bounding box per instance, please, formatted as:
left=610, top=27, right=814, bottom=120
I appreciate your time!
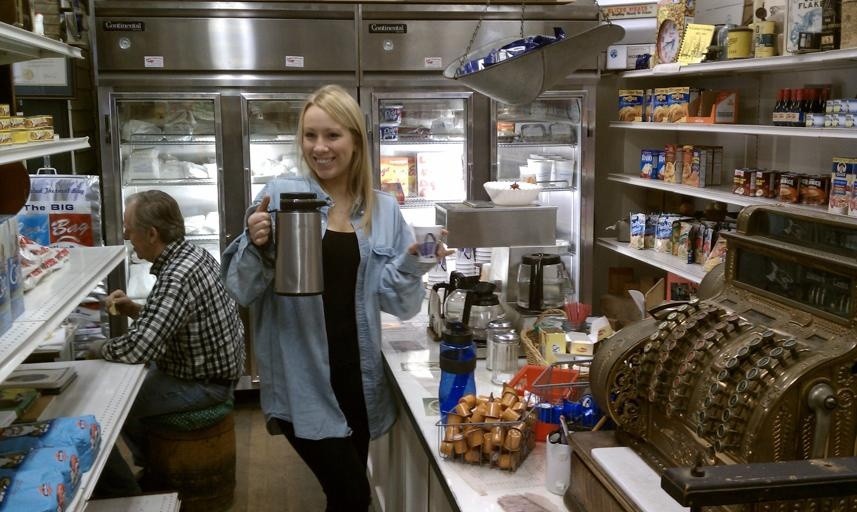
left=481, top=182, right=545, bottom=205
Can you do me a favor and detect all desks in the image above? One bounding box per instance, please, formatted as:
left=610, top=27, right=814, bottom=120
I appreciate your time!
left=381, top=284, right=607, bottom=511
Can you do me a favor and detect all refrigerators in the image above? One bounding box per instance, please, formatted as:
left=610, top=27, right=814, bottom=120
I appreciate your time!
left=88, top=0, right=359, bottom=392
left=359, top=3, right=603, bottom=311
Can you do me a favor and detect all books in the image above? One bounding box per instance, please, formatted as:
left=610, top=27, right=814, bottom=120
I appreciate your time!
left=35, top=328, right=66, bottom=353
left=0, top=388, right=56, bottom=428
left=0, top=366, right=78, bottom=394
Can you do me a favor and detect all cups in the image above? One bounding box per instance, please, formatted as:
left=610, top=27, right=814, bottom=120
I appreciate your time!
left=518, top=154, right=575, bottom=188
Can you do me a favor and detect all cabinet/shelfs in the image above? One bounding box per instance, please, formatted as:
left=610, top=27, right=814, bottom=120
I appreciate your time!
left=0, top=20, right=182, bottom=511
left=594, top=60, right=857, bottom=320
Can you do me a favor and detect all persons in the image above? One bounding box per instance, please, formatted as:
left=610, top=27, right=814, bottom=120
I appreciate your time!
left=219, top=84, right=456, bottom=512
left=78, top=191, right=246, bottom=499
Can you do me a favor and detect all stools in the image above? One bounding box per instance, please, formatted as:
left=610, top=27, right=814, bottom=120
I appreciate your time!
left=152, top=397, right=237, bottom=512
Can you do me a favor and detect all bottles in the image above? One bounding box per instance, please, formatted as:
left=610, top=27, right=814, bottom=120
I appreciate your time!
left=491, top=332, right=520, bottom=384
left=784, top=88, right=790, bottom=126
left=790, top=88, right=797, bottom=126
left=809, top=88, right=823, bottom=112
left=438, top=319, right=477, bottom=428
left=823, top=84, right=831, bottom=112
left=797, top=88, right=807, bottom=126
left=485, top=318, right=513, bottom=370
left=774, top=89, right=783, bottom=125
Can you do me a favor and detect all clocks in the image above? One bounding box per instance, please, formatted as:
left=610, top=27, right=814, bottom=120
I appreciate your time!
left=650, top=4, right=686, bottom=65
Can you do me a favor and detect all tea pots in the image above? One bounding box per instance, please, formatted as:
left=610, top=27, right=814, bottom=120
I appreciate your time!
left=461, top=282, right=507, bottom=343
left=515, top=252, right=570, bottom=314
left=259, top=193, right=327, bottom=298
left=440, top=270, right=482, bottom=331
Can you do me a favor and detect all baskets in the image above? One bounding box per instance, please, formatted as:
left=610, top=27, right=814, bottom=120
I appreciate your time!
left=520, top=308, right=567, bottom=366
